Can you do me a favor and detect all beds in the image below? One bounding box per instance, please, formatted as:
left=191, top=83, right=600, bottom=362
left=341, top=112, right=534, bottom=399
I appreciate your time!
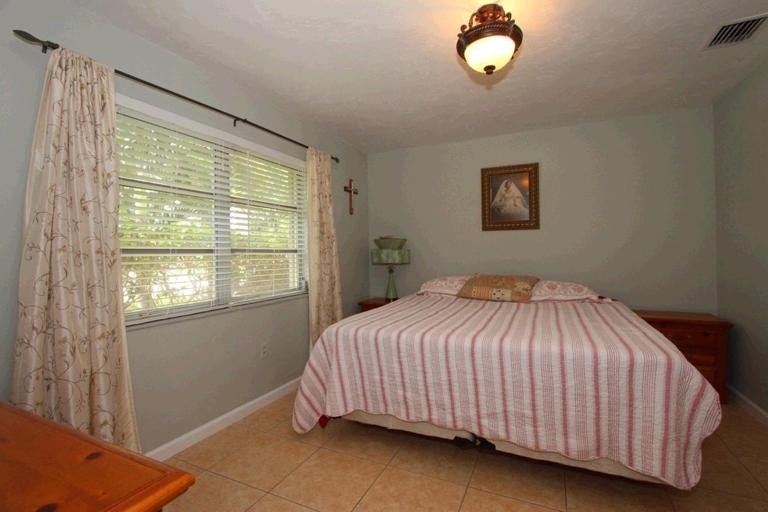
left=287, top=286, right=725, bottom=495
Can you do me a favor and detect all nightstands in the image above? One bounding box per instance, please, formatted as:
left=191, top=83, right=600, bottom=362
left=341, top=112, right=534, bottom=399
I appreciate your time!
left=356, top=295, right=401, bottom=313
left=630, top=308, right=734, bottom=405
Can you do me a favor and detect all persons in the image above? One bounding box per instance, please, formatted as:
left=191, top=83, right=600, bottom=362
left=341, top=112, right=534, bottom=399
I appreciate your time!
left=489, top=178, right=529, bottom=222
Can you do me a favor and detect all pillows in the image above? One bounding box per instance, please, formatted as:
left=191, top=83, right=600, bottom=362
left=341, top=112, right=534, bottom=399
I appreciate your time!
left=457, top=271, right=542, bottom=304
left=529, top=278, right=602, bottom=303
left=415, top=273, right=474, bottom=299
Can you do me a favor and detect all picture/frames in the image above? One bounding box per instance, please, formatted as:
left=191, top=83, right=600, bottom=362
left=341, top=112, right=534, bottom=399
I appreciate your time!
left=478, top=161, right=541, bottom=234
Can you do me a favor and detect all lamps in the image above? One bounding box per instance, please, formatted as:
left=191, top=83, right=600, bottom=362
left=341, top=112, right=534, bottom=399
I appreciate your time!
left=369, top=234, right=414, bottom=303
left=453, top=2, right=524, bottom=79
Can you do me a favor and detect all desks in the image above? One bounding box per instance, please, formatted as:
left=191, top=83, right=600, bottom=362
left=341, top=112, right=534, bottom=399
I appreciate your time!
left=0, top=399, right=198, bottom=511
